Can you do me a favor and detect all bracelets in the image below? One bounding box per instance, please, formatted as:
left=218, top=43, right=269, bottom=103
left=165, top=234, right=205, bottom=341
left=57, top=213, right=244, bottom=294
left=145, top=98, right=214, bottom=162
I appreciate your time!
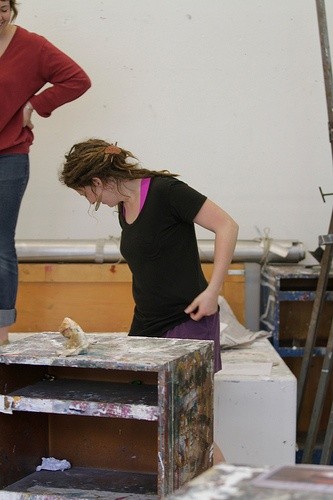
left=25, top=104, right=33, bottom=112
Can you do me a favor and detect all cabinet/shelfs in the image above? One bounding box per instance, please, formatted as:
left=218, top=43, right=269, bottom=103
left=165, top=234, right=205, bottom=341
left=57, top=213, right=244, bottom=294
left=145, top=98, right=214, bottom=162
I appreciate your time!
left=259, top=264, right=333, bottom=447
left=0, top=332, right=215, bottom=500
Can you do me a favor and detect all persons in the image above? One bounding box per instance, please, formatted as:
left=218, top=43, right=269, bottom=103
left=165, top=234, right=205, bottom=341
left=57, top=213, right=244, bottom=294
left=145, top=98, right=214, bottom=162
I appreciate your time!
left=1, top=0, right=92, bottom=344
left=58, top=139, right=240, bottom=467
